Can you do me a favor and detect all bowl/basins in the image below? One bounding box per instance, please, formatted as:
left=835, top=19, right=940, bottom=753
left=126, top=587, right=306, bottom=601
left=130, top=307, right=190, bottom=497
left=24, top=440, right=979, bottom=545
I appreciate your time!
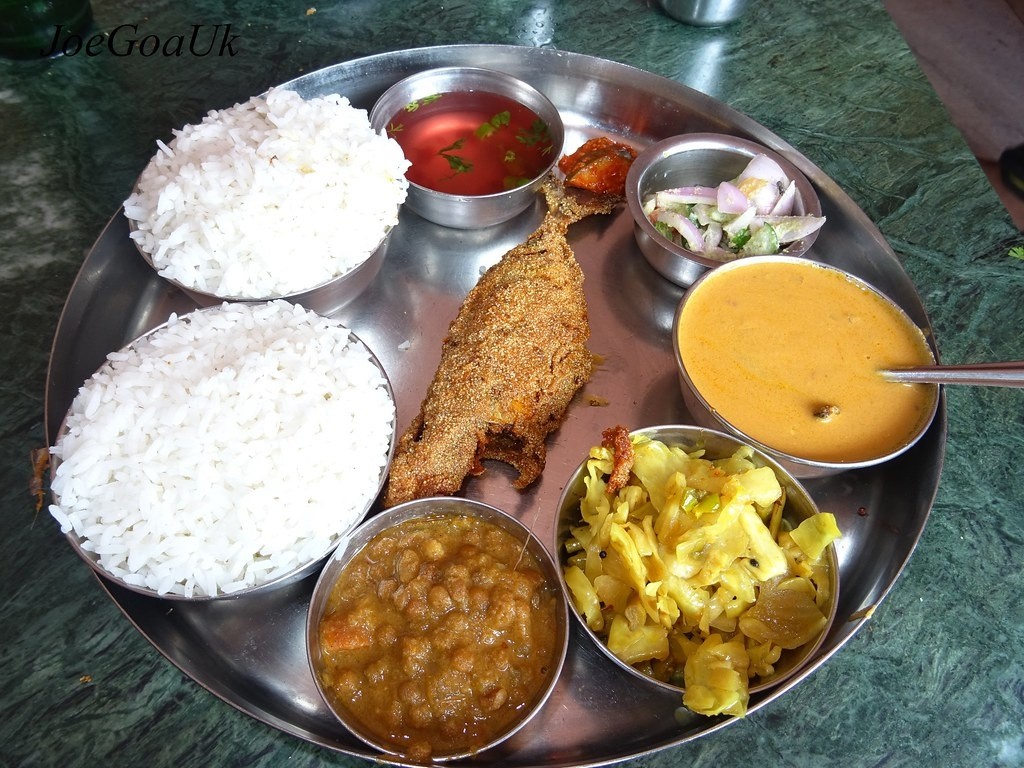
left=50, top=302, right=398, bottom=600
left=673, top=255, right=939, bottom=480
left=626, top=132, right=823, bottom=291
left=554, top=424, right=841, bottom=697
left=128, top=152, right=398, bottom=319
left=305, top=497, right=568, bottom=763
left=369, top=65, right=565, bottom=230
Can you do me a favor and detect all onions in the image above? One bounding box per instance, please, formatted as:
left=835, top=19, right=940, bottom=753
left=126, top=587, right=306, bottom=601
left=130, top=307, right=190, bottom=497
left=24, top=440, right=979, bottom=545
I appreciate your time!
left=655, top=152, right=828, bottom=251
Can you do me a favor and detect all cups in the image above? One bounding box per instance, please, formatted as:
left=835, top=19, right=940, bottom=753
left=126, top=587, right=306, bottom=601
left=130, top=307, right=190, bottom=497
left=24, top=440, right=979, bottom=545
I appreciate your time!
left=647, top=0, right=756, bottom=30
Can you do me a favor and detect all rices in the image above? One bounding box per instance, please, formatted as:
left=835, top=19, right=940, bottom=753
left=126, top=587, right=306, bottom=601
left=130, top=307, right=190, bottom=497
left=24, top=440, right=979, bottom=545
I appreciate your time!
left=121, top=86, right=413, bottom=299
left=46, top=299, right=396, bottom=598
